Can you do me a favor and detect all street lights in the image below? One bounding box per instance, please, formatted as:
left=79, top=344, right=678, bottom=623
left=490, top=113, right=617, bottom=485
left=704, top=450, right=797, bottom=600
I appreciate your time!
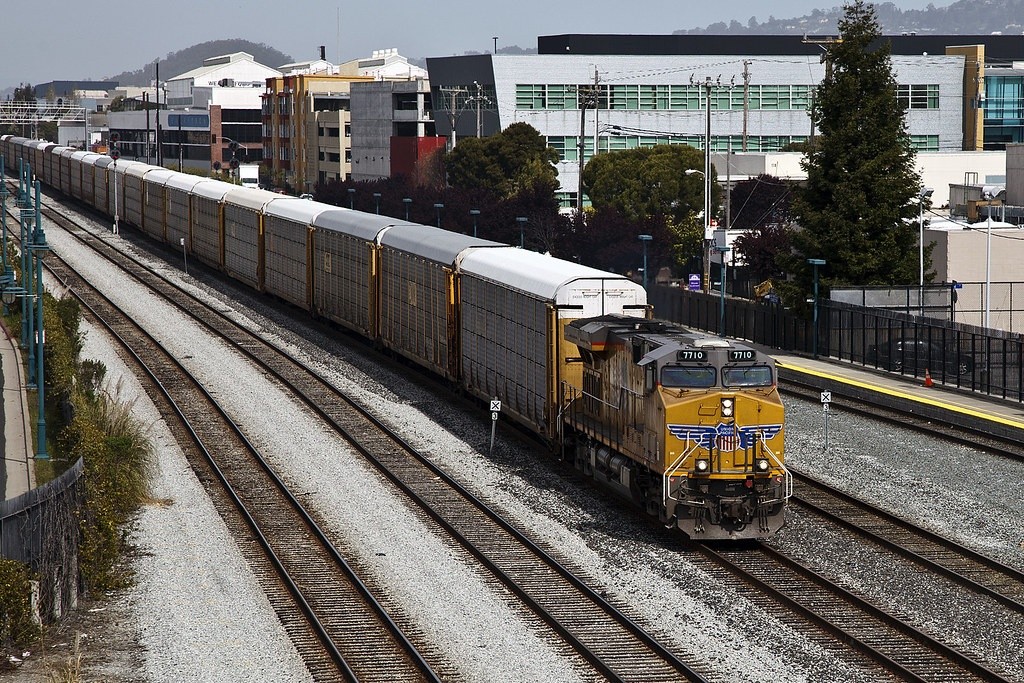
left=981, top=184, right=1007, bottom=328
left=715, top=245, right=731, bottom=337
left=685, top=167, right=710, bottom=293
left=470, top=209, right=481, bottom=237
left=402, top=197, right=413, bottom=221
left=516, top=217, right=528, bottom=249
left=434, top=203, right=444, bottom=229
left=806, top=258, right=827, bottom=359
left=305, top=180, right=311, bottom=199
left=347, top=188, right=357, bottom=209
left=920, top=186, right=934, bottom=314
left=637, top=234, right=652, bottom=291
left=374, top=192, right=381, bottom=215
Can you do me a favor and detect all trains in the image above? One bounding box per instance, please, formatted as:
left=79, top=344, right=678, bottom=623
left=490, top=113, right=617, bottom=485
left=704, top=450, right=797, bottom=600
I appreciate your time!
left=0, top=132, right=790, bottom=545
